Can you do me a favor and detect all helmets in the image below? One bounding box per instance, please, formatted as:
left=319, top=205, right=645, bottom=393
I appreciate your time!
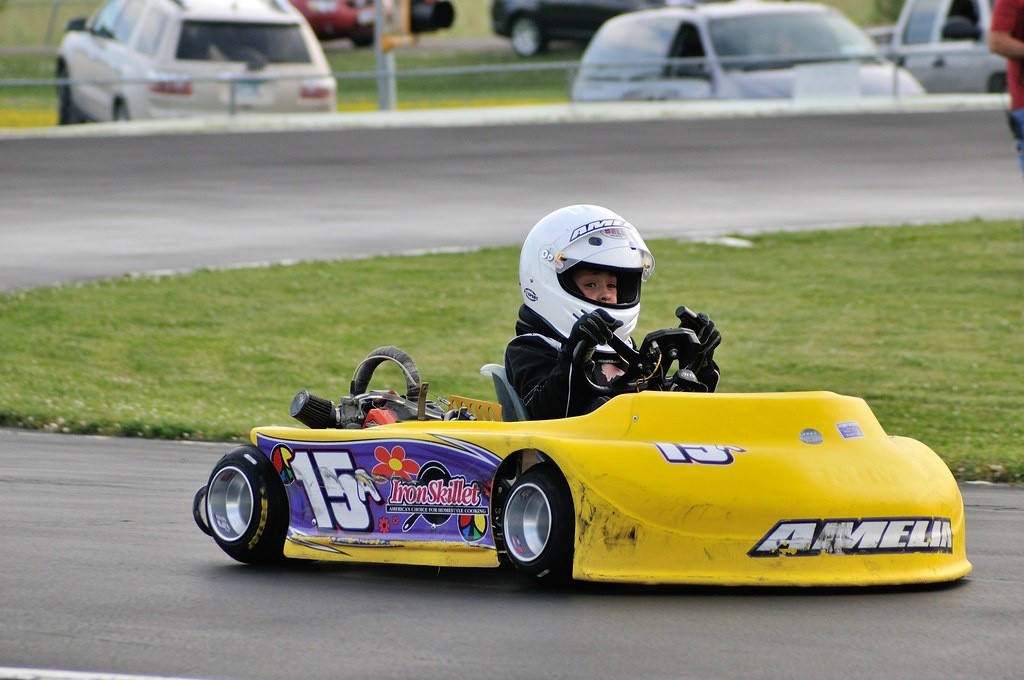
left=519, top=205, right=657, bottom=353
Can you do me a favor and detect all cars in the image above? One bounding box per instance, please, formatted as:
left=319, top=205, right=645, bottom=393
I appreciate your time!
left=490, top=0, right=739, bottom=56
left=568, top=0, right=928, bottom=105
left=53, top=0, right=339, bottom=126
left=287, top=0, right=454, bottom=46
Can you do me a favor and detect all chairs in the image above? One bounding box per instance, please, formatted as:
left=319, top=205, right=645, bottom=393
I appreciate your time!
left=480, top=363, right=529, bottom=422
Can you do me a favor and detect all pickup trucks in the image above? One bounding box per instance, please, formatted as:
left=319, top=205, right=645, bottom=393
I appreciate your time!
left=847, top=0, right=1010, bottom=96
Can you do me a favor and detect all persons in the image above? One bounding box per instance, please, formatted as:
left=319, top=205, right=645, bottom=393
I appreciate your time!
left=988, top=0, right=1024, bottom=179
left=505, top=204, right=722, bottom=421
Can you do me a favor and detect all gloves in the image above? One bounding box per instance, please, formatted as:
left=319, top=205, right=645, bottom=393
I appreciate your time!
left=564, top=308, right=623, bottom=376
left=678, top=311, right=722, bottom=376
